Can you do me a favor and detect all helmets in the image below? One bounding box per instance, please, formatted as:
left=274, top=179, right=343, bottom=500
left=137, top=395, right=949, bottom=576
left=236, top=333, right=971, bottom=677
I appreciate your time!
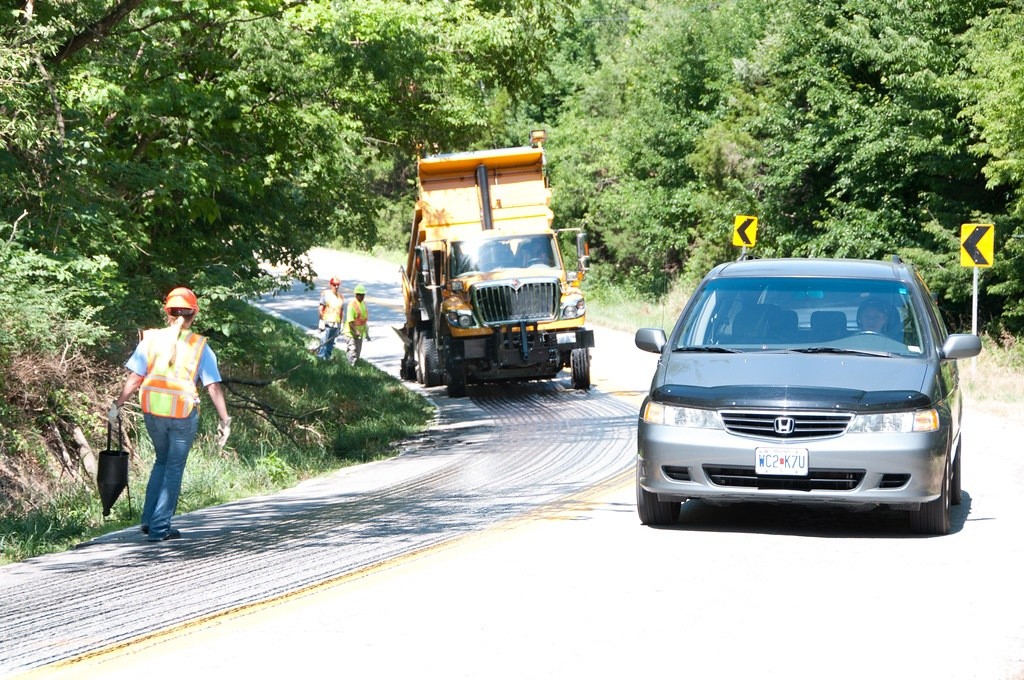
left=856, top=297, right=889, bottom=329
left=330, top=277, right=340, bottom=285
left=353, top=284, right=367, bottom=294
left=164, top=287, right=199, bottom=315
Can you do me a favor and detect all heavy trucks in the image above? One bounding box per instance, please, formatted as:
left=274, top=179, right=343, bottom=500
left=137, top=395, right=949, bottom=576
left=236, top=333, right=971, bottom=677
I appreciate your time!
left=390, top=125, right=595, bottom=395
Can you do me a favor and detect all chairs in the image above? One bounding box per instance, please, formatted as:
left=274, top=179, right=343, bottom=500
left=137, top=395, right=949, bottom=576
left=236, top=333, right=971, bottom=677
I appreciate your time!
left=717, top=302, right=900, bottom=344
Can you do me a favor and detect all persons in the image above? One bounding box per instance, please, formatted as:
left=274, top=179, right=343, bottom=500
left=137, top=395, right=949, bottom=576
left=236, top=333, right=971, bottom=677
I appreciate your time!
left=317, top=276, right=345, bottom=359
left=522, top=238, right=551, bottom=266
left=345, top=282, right=370, bottom=366
left=108, top=286, right=232, bottom=542
left=855, top=300, right=892, bottom=332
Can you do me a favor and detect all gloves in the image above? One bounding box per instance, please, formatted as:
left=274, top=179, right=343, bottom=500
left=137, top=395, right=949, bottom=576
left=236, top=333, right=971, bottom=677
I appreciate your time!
left=107, top=400, right=124, bottom=427
left=217, top=417, right=231, bottom=447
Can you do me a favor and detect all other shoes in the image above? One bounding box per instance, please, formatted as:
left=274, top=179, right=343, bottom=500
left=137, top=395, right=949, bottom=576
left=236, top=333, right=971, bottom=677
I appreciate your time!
left=141, top=525, right=181, bottom=542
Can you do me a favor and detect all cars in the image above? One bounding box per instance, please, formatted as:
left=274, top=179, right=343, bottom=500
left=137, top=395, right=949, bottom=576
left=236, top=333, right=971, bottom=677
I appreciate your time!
left=634, top=250, right=981, bottom=538
